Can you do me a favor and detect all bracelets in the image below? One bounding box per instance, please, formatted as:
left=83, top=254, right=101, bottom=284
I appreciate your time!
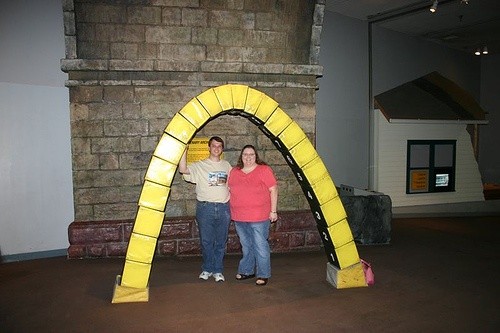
left=270, top=211, right=277, bottom=214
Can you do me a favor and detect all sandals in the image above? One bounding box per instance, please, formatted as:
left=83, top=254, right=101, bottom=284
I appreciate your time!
left=235, top=274, right=255, bottom=280
left=256, top=278, right=268, bottom=286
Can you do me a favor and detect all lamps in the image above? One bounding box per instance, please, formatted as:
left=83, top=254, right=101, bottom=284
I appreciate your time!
left=474, top=46, right=490, bottom=56
left=428, top=0, right=442, bottom=14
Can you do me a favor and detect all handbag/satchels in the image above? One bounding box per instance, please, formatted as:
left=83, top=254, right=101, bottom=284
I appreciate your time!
left=360, top=260, right=374, bottom=286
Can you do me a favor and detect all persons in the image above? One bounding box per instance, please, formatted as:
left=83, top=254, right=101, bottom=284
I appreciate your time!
left=227, top=145, right=277, bottom=286
left=178, top=136, right=232, bottom=282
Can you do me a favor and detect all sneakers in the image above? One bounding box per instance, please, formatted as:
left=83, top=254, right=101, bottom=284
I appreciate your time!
left=213, top=273, right=225, bottom=282
left=199, top=271, right=212, bottom=281
